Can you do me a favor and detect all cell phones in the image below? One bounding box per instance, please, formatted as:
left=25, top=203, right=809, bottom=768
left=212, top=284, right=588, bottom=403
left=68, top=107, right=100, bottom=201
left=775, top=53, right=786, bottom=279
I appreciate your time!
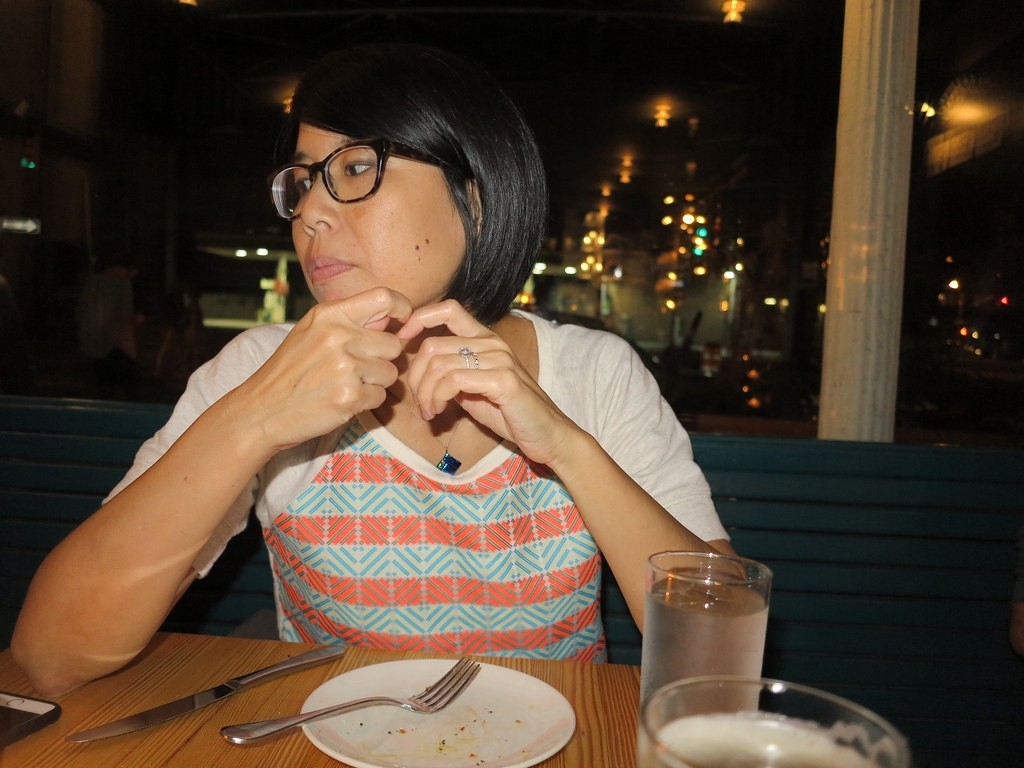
left=0, top=690, right=63, bottom=748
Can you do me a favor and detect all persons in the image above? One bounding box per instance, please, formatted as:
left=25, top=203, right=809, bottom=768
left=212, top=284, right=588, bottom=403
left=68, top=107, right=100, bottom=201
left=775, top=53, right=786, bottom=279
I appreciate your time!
left=11, top=45, right=764, bottom=699
left=78, top=237, right=206, bottom=402
left=1010, top=528, right=1024, bottom=654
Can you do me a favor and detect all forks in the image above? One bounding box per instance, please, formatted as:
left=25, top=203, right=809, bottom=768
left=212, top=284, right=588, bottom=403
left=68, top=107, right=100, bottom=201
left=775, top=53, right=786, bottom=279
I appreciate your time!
left=220, top=655, right=481, bottom=745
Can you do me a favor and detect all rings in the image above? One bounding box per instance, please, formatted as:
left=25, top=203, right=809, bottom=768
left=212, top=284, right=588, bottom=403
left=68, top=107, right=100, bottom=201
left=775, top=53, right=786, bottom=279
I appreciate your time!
left=459, top=347, right=479, bottom=370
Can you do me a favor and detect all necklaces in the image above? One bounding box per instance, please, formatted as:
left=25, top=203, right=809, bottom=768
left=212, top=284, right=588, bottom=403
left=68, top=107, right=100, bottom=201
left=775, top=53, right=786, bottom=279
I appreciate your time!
left=385, top=389, right=463, bottom=475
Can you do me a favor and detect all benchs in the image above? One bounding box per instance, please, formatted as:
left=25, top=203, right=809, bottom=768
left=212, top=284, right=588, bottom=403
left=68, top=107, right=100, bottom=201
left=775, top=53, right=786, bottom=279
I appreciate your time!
left=0, top=393, right=1024, bottom=768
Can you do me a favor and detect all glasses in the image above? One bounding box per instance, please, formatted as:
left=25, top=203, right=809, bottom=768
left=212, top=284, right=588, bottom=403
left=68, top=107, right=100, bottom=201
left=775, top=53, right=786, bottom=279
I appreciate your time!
left=265, top=137, right=469, bottom=222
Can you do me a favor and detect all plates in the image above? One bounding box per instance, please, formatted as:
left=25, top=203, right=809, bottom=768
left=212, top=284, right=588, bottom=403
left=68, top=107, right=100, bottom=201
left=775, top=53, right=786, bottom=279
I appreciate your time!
left=299, top=658, right=576, bottom=768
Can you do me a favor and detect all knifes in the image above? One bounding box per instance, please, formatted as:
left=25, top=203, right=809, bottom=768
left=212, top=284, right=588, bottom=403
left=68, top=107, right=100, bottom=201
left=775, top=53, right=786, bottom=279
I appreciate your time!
left=64, top=644, right=346, bottom=743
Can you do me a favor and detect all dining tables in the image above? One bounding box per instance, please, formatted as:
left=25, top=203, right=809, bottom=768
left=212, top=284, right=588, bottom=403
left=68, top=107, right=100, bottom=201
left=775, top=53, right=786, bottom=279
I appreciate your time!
left=0, top=630, right=641, bottom=768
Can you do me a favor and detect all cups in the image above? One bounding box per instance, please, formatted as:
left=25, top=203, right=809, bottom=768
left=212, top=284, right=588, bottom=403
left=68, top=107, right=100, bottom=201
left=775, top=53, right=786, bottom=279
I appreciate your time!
left=639, top=674, right=911, bottom=768
left=638, top=549, right=774, bottom=733
